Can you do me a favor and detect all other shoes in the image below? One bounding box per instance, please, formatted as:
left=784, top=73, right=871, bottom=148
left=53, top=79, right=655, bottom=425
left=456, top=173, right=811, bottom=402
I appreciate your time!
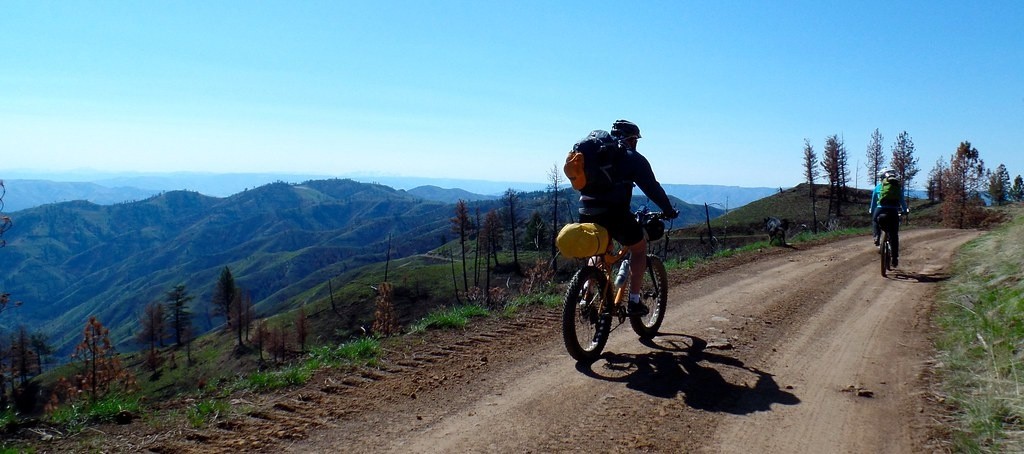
left=579, top=292, right=599, bottom=324
left=873, top=235, right=880, bottom=246
left=891, top=254, right=899, bottom=268
left=627, top=299, right=650, bottom=316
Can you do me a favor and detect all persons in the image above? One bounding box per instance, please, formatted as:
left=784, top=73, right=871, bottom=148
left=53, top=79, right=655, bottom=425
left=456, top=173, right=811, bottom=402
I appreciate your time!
left=869, top=168, right=908, bottom=267
left=579, top=119, right=679, bottom=318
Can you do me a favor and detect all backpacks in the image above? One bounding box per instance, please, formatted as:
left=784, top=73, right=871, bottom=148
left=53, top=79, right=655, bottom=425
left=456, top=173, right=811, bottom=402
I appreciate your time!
left=879, top=178, right=902, bottom=206
left=573, top=128, right=636, bottom=201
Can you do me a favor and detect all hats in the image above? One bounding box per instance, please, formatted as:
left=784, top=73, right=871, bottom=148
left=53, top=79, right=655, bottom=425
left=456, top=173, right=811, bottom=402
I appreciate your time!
left=564, top=151, right=585, bottom=190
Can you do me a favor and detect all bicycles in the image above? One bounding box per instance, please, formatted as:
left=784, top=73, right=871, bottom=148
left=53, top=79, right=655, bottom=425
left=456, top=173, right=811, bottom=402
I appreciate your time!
left=871, top=209, right=909, bottom=274
left=562, top=206, right=682, bottom=360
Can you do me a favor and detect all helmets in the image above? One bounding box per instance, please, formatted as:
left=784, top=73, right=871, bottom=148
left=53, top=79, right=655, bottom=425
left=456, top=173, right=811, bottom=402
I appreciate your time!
left=876, top=168, right=897, bottom=180
left=611, top=118, right=642, bottom=141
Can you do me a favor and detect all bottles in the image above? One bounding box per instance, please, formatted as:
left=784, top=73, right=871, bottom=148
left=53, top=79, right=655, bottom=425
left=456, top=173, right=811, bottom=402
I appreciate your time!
left=887, top=241, right=892, bottom=256
left=614, top=260, right=630, bottom=287
